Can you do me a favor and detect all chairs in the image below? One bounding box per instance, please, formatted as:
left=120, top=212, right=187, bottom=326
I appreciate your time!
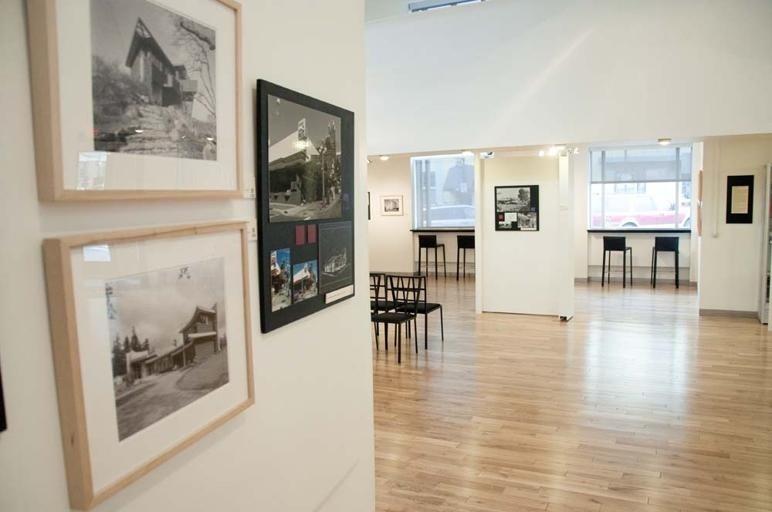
left=652, top=236, right=679, bottom=290
left=601, top=235, right=633, bottom=288
left=418, top=233, right=448, bottom=280
left=457, top=234, right=475, bottom=279
left=369, top=271, right=444, bottom=365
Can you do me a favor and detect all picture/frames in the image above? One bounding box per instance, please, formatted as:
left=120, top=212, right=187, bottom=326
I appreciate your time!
left=494, top=186, right=542, bottom=232
left=381, top=194, right=403, bottom=217
left=41, top=222, right=255, bottom=510
left=726, top=172, right=754, bottom=224
left=24, top=0, right=245, bottom=202
left=256, top=76, right=359, bottom=337
